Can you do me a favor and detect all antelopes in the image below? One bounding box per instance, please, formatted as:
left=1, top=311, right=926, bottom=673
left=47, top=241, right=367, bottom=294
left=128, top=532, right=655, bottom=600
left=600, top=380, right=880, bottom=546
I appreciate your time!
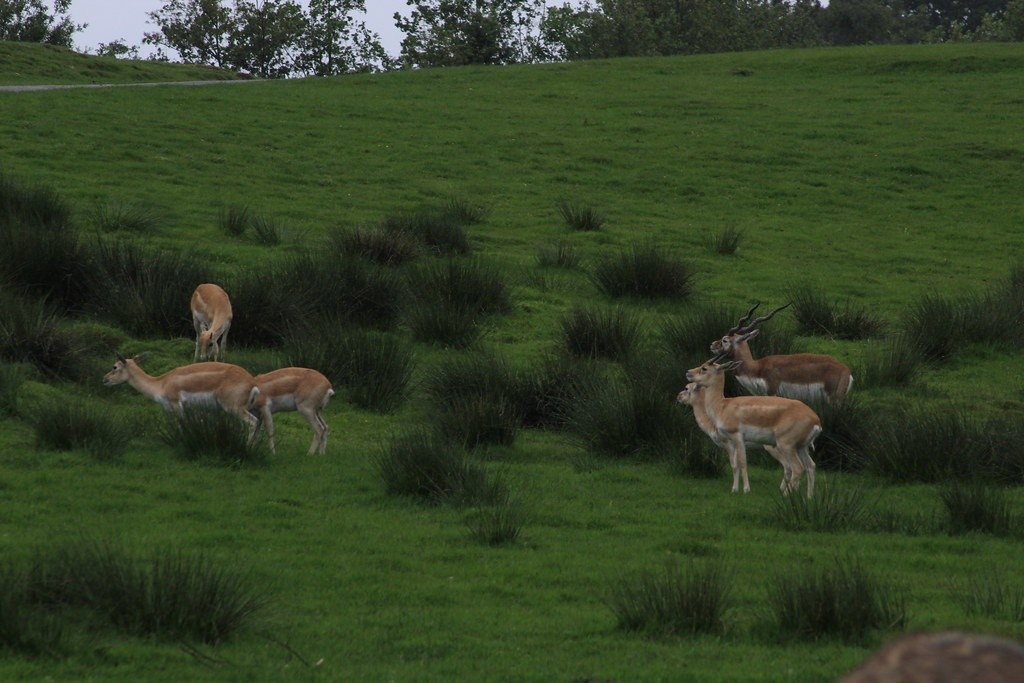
left=676, top=383, right=792, bottom=493
left=684, top=349, right=823, bottom=499
left=249, top=367, right=336, bottom=457
left=102, top=352, right=260, bottom=450
left=187, top=283, right=233, bottom=362
left=710, top=301, right=854, bottom=400
